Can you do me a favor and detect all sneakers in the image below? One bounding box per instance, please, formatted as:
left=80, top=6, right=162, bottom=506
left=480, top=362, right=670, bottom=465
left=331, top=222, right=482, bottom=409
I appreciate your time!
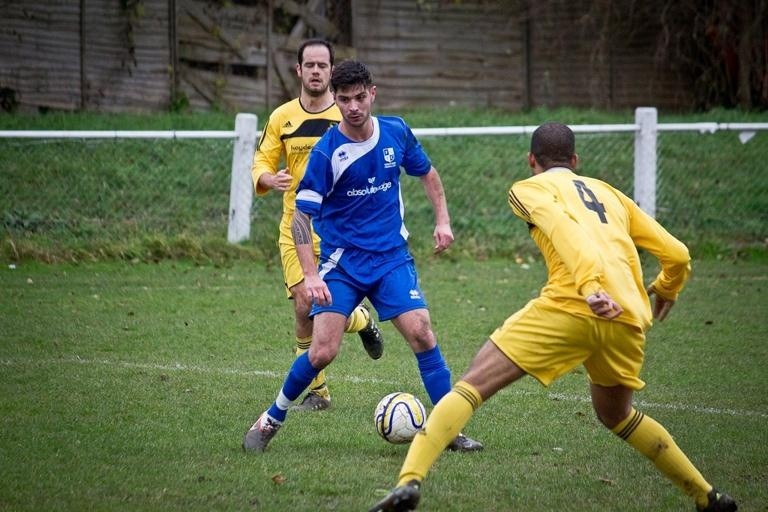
left=357, top=300, right=384, bottom=360
left=242, top=411, right=283, bottom=455
left=290, top=392, right=332, bottom=412
left=696, top=488, right=740, bottom=512
left=365, top=479, right=422, bottom=512
left=446, top=432, right=484, bottom=452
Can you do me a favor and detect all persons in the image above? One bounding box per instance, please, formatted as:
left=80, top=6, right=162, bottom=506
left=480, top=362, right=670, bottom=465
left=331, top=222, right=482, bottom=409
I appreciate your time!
left=251, top=39, right=384, bottom=413
left=369, top=123, right=738, bottom=512
left=240, top=60, right=482, bottom=452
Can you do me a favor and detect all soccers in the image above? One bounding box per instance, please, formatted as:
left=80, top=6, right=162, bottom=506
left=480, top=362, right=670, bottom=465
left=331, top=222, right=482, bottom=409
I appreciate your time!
left=374, top=391, right=426, bottom=443
